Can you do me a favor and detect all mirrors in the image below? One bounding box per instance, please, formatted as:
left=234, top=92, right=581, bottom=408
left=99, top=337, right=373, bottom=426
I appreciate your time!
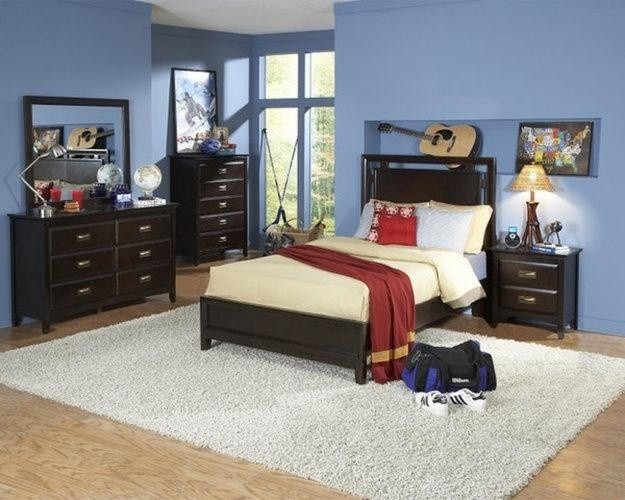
left=23, top=96, right=131, bottom=214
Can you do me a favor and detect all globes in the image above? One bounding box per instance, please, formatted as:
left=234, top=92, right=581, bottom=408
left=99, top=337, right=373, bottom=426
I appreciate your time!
left=133, top=164, right=162, bottom=200
left=96, top=163, right=124, bottom=190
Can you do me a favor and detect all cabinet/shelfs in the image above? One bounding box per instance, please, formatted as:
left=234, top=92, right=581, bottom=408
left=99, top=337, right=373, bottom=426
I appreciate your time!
left=7, top=197, right=176, bottom=334
left=167, top=153, right=249, bottom=267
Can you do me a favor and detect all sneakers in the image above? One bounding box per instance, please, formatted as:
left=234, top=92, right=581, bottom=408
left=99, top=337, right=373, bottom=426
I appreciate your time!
left=415, top=391, right=450, bottom=417
left=443, top=388, right=490, bottom=413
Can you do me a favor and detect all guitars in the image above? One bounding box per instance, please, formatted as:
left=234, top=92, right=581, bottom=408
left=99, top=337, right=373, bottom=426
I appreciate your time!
left=378, top=123, right=481, bottom=169
left=67, top=127, right=114, bottom=148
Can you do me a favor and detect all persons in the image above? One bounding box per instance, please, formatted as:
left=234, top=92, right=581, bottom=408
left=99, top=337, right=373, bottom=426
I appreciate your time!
left=176, top=91, right=211, bottom=126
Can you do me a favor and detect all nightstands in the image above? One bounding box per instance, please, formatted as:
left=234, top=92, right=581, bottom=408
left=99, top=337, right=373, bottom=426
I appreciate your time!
left=487, top=242, right=583, bottom=339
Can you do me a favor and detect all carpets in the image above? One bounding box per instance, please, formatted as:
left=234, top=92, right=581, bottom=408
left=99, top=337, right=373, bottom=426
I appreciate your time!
left=1, top=303, right=625, bottom=500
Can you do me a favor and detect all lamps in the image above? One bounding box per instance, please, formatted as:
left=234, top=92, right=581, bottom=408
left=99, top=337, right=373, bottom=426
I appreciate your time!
left=507, top=162, right=555, bottom=246
left=17, top=143, right=67, bottom=215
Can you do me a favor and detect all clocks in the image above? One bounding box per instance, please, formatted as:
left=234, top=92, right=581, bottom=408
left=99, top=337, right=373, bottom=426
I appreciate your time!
left=503, top=233, right=522, bottom=249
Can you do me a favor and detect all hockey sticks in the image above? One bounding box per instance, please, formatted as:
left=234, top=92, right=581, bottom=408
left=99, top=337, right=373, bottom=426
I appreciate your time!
left=263, top=138, right=300, bottom=232
left=264, top=128, right=292, bottom=230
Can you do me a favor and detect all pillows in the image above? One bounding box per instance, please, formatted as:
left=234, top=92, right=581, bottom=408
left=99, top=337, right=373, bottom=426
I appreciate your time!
left=34, top=180, right=90, bottom=202
left=353, top=199, right=493, bottom=255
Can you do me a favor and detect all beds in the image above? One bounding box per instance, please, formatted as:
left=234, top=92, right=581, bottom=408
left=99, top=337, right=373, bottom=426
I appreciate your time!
left=199, top=153, right=497, bottom=385
left=33, top=149, right=110, bottom=187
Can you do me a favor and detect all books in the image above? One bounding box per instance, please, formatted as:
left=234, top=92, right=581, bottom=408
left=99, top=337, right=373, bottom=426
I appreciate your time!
left=532, top=242, right=571, bottom=254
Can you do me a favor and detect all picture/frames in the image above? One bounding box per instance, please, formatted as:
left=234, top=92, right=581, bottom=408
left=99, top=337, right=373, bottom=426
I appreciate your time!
left=33, top=126, right=63, bottom=158
left=213, top=126, right=228, bottom=144
left=515, top=120, right=594, bottom=176
left=171, top=67, right=218, bottom=153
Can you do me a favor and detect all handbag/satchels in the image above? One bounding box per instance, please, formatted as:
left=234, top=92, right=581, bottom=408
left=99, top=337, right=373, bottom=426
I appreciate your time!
left=402, top=340, right=496, bottom=393
